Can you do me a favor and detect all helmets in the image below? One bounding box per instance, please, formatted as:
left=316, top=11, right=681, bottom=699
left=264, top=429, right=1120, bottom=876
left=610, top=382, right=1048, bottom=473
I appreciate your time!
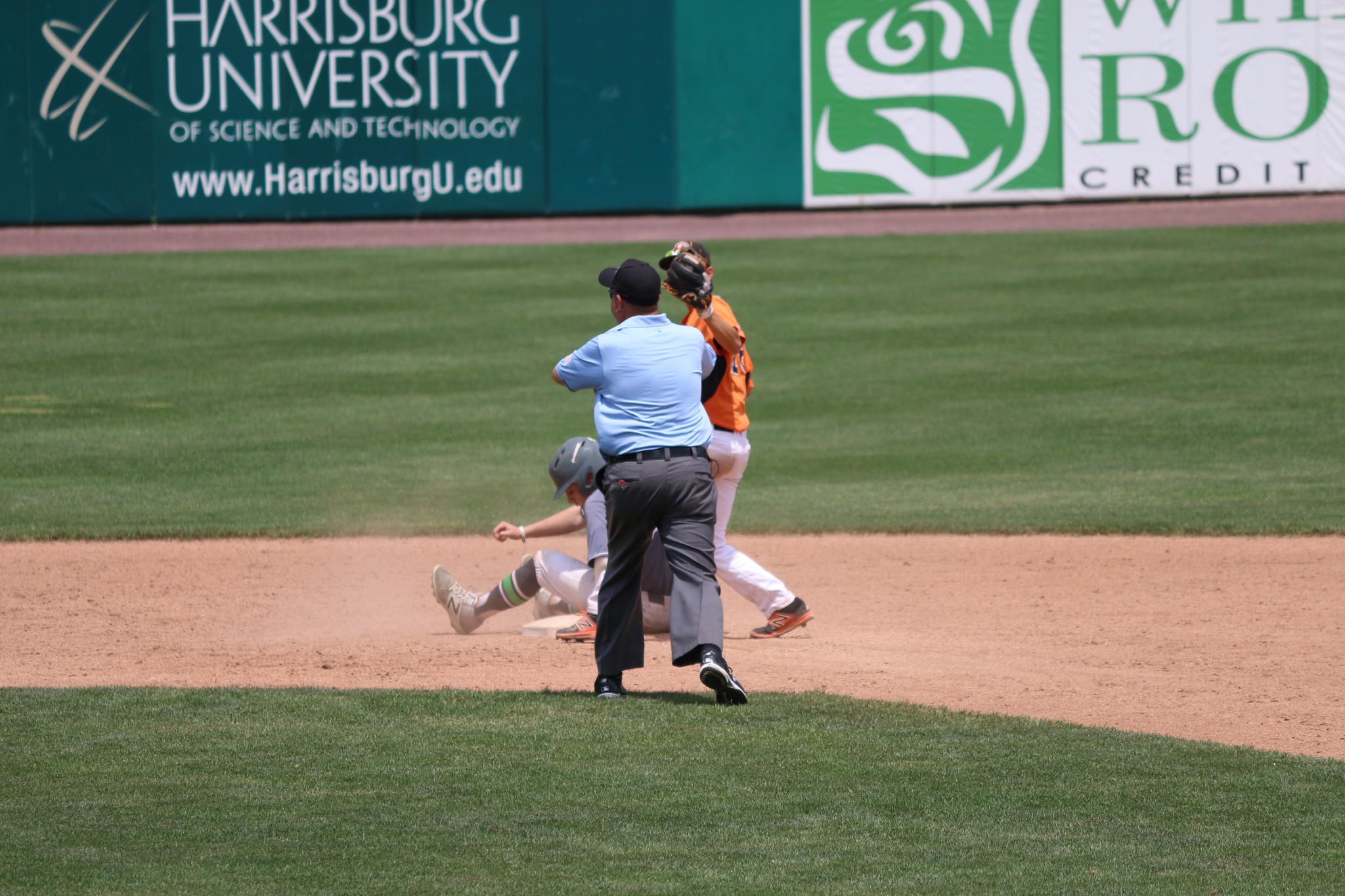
left=548, top=435, right=609, bottom=502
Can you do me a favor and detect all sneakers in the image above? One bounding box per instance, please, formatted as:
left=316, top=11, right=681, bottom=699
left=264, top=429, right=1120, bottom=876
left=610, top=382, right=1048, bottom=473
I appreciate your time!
left=555, top=604, right=600, bottom=642
left=430, top=566, right=484, bottom=636
left=594, top=673, right=628, bottom=700
left=522, top=553, right=556, bottom=618
left=699, top=650, right=748, bottom=706
left=751, top=597, right=814, bottom=639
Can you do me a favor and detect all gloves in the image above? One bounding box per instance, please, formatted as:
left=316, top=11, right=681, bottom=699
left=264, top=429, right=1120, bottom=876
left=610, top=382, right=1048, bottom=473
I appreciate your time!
left=664, top=253, right=713, bottom=321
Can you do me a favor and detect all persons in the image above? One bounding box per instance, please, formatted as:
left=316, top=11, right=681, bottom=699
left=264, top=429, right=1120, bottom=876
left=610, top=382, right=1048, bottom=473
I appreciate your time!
left=429, top=436, right=673, bottom=634
left=555, top=240, right=814, bottom=642
left=551, top=257, right=748, bottom=706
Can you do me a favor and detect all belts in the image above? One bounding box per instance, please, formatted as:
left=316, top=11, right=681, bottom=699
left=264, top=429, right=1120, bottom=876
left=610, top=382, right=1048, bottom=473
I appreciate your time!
left=648, top=591, right=666, bottom=604
left=607, top=446, right=710, bottom=464
left=715, top=423, right=732, bottom=433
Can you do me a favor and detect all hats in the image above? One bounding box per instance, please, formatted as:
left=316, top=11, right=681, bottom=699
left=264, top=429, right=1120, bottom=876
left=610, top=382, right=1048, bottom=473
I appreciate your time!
left=598, top=258, right=661, bottom=306
left=657, top=241, right=711, bottom=272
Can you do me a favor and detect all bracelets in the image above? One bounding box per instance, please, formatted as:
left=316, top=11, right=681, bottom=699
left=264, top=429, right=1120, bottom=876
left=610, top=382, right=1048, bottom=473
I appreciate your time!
left=520, top=525, right=526, bottom=543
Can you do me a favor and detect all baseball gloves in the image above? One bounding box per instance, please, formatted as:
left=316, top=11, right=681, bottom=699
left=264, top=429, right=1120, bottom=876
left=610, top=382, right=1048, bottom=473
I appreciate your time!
left=660, top=250, right=715, bottom=314
